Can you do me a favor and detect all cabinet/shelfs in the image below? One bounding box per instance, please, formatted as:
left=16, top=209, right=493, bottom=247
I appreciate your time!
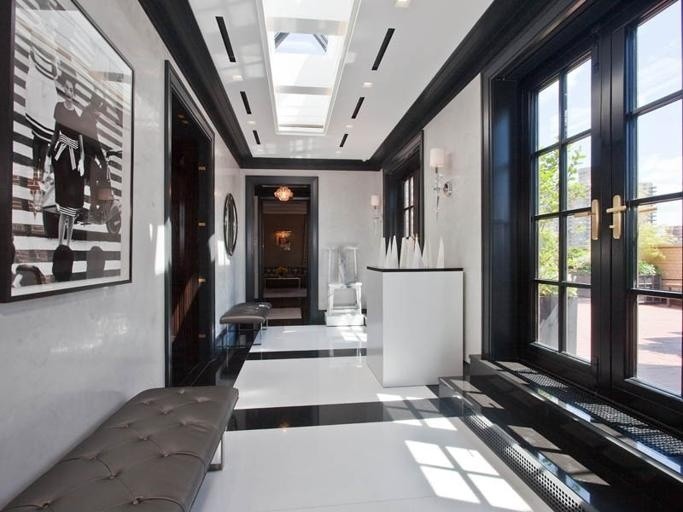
left=366, top=265, right=464, bottom=388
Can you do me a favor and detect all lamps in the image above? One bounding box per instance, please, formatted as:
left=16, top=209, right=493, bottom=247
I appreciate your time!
left=371, top=196, right=380, bottom=219
left=430, top=149, right=452, bottom=209
left=274, top=185, right=294, bottom=202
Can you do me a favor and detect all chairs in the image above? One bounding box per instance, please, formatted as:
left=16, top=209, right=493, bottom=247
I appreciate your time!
left=326, top=246, right=363, bottom=315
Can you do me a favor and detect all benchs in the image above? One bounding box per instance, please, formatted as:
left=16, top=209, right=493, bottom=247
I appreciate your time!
left=0, top=387, right=238, bottom=511
left=220, top=302, right=272, bottom=349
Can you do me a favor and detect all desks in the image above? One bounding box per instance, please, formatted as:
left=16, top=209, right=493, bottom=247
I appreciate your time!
left=264, top=277, right=301, bottom=289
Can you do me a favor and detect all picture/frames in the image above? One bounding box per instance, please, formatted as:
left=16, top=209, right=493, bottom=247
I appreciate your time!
left=1, top=1, right=135, bottom=302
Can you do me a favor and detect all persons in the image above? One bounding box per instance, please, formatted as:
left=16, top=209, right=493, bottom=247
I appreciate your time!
left=46, top=78, right=89, bottom=248
left=76, top=96, right=109, bottom=186
left=23, top=14, right=61, bottom=181
